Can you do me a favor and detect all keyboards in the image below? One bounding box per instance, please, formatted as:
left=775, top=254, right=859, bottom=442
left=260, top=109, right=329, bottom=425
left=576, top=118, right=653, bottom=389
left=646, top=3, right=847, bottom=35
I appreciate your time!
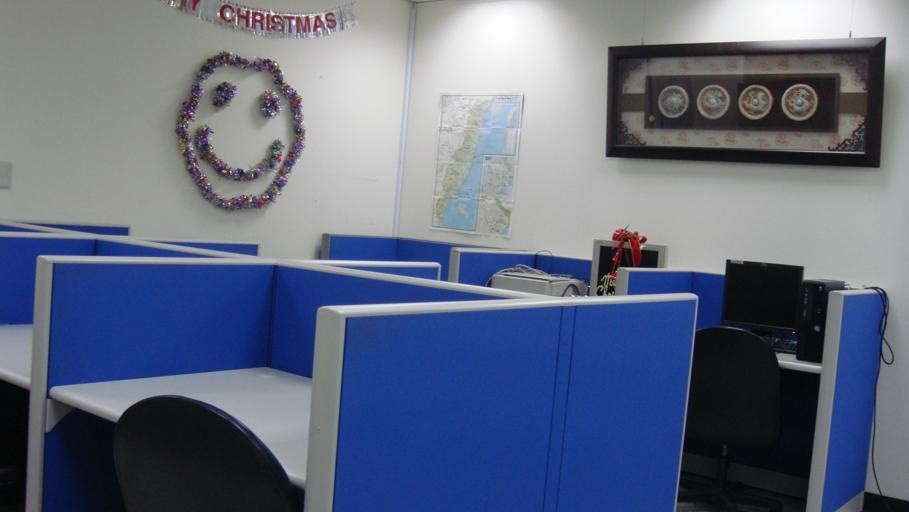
left=763, top=336, right=798, bottom=354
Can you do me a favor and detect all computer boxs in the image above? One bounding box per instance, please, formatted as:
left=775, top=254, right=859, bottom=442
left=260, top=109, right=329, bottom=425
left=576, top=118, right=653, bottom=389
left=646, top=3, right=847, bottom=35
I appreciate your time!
left=796, top=279, right=844, bottom=363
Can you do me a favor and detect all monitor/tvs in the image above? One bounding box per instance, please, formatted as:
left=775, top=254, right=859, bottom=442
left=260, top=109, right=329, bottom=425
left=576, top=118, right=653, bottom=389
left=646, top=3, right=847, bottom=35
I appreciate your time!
left=722, top=259, right=804, bottom=338
left=589, top=239, right=667, bottom=296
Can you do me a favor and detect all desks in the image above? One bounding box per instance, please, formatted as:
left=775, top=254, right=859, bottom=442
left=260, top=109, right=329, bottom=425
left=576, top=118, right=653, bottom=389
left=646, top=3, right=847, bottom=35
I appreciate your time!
left=1, top=310, right=312, bottom=488
left=777, top=350, right=826, bottom=377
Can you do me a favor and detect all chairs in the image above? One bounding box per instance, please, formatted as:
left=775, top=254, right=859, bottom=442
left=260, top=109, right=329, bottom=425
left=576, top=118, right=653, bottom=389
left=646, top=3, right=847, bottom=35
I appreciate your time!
left=678, top=326, right=783, bottom=511
left=113, top=395, right=302, bottom=512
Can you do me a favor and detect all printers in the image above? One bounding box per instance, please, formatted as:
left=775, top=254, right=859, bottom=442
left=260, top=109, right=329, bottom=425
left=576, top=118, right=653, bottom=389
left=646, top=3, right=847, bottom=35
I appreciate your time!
left=491, top=271, right=587, bottom=297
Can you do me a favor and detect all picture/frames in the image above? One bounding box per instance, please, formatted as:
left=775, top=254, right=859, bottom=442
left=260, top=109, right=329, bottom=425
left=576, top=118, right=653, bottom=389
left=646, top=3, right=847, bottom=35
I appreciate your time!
left=605, top=37, right=886, bottom=168
